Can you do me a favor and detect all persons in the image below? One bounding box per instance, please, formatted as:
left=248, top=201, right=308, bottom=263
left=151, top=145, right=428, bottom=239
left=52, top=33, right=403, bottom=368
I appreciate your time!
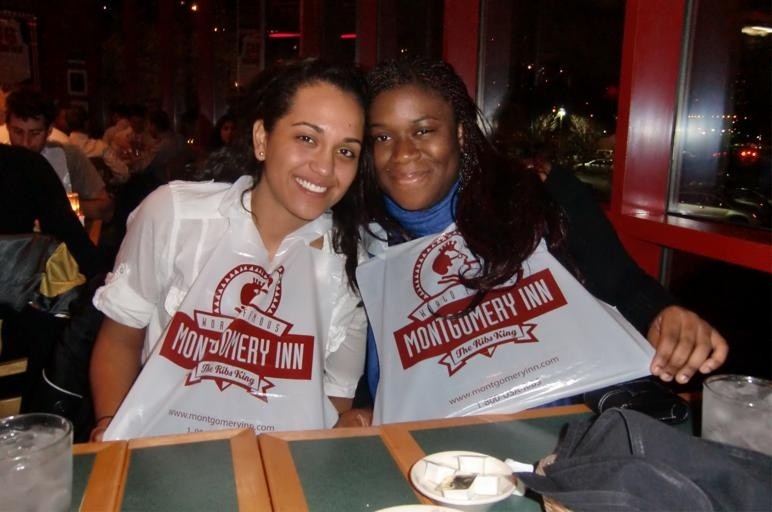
left=331, top=47, right=732, bottom=427
left=1, top=92, right=237, bottom=403
left=87, top=56, right=368, bottom=442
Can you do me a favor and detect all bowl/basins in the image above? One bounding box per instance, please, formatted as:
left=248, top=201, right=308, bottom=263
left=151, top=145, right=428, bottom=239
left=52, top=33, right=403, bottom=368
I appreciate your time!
left=410, top=450, right=517, bottom=511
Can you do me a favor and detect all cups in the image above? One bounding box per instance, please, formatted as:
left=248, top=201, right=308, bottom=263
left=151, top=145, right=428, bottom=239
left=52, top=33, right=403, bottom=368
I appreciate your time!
left=0, top=412, right=76, bottom=511
left=700, top=375, right=772, bottom=457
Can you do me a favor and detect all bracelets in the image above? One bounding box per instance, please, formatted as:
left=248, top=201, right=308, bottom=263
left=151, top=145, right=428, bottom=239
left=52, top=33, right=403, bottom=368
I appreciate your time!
left=93, top=415, right=113, bottom=426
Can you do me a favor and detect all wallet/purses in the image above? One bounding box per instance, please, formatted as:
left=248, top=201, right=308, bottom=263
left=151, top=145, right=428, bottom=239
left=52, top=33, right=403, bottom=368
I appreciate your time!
left=584, top=380, right=690, bottom=424
